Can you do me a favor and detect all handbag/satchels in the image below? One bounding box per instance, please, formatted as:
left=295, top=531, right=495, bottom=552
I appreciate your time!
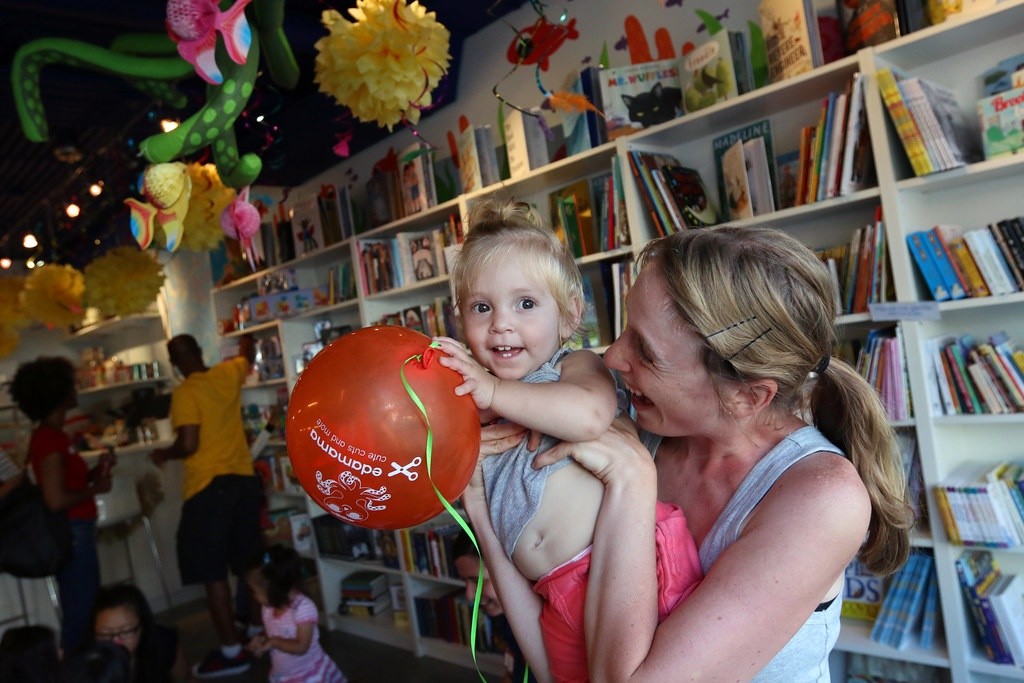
left=0, top=467, right=76, bottom=578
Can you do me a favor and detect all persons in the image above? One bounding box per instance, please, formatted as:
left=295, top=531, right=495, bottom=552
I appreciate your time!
left=0, top=448, right=21, bottom=495
left=149, top=334, right=265, bottom=677
left=9, top=356, right=116, bottom=664
left=0, top=581, right=189, bottom=683
left=244, top=544, right=348, bottom=683
left=459, top=228, right=912, bottom=682
left=429, top=195, right=704, bottom=683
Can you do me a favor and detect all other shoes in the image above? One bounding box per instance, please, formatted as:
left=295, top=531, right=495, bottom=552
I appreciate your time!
left=192, top=645, right=250, bottom=678
left=234, top=616, right=265, bottom=638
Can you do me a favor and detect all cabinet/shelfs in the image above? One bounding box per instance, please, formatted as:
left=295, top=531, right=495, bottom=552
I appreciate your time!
left=210, top=0, right=1024, bottom=683
left=63, top=285, right=185, bottom=458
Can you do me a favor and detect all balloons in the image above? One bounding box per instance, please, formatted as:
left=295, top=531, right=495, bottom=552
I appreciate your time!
left=285, top=325, right=480, bottom=530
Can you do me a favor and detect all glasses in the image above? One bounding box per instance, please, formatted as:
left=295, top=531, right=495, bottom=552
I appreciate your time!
left=94, top=620, right=144, bottom=643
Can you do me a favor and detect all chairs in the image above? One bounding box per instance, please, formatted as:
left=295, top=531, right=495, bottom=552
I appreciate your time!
left=92, top=467, right=181, bottom=622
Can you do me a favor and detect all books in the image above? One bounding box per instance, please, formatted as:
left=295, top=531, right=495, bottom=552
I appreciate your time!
left=225, top=105, right=547, bottom=330
left=302, top=296, right=454, bottom=372
left=876, top=50, right=1024, bottom=177
left=259, top=507, right=505, bottom=656
left=221, top=336, right=306, bottom=498
left=547, top=0, right=911, bottom=259
left=831, top=326, right=1024, bottom=683
left=906, top=214, right=1024, bottom=300
left=562, top=260, right=635, bottom=350
left=813, top=205, right=897, bottom=315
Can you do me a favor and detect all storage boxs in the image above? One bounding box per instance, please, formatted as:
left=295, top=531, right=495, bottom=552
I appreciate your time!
left=250, top=287, right=328, bottom=323
left=312, top=512, right=372, bottom=563
